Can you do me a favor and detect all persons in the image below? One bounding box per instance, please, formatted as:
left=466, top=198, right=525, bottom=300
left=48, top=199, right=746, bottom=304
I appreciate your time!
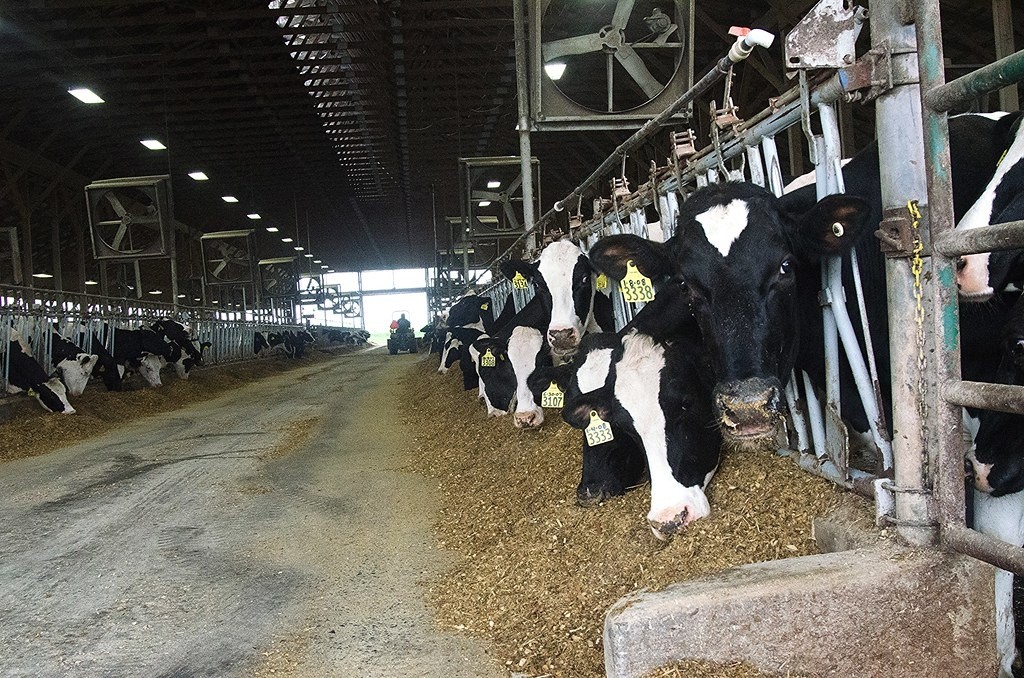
left=397, top=314, right=410, bottom=333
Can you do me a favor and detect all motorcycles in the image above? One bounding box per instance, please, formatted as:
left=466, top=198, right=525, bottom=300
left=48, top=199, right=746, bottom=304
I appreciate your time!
left=388, top=329, right=418, bottom=355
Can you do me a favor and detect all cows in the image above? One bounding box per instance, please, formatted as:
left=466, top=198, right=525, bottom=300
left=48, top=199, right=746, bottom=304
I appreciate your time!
left=253, top=329, right=370, bottom=358
left=0, top=315, right=211, bottom=415
left=587, top=111, right=1024, bottom=498
left=438, top=239, right=616, bottom=430
left=527, top=279, right=725, bottom=543
